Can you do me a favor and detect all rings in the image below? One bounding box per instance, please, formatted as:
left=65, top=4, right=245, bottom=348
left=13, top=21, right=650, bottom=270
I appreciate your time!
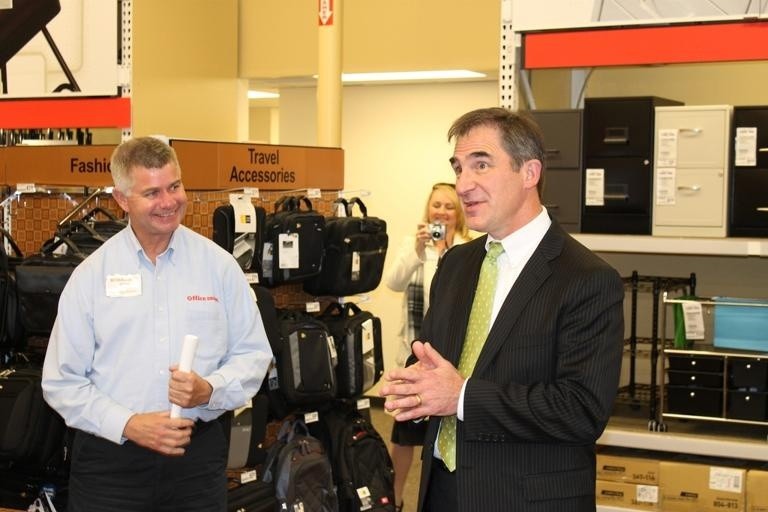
left=416, top=395, right=422, bottom=408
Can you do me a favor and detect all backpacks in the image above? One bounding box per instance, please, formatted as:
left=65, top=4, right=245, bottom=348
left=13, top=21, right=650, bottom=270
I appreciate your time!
left=261, top=408, right=395, bottom=512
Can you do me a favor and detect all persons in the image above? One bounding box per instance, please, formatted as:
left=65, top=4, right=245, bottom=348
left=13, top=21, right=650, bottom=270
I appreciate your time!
left=388, top=184, right=475, bottom=512
left=380, top=107, right=625, bottom=512
left=41, top=138, right=274, bottom=512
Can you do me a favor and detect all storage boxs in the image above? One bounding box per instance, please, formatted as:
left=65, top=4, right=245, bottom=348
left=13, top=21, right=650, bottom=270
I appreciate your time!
left=596, top=453, right=767, bottom=512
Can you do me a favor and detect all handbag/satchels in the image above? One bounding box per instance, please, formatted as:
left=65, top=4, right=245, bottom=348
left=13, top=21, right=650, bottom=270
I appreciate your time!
left=0, top=207, right=126, bottom=511
left=266, top=302, right=384, bottom=404
left=211, top=193, right=388, bottom=297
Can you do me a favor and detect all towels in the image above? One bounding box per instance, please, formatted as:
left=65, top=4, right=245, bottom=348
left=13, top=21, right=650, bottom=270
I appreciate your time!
left=674, top=292, right=705, bottom=352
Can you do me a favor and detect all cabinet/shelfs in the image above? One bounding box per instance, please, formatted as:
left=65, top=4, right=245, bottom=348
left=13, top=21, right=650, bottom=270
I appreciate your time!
left=654, top=104, right=737, bottom=237
left=665, top=350, right=767, bottom=423
left=587, top=97, right=685, bottom=236
left=727, top=106, right=768, bottom=237
left=501, top=0, right=768, bottom=511
left=515, top=107, right=584, bottom=237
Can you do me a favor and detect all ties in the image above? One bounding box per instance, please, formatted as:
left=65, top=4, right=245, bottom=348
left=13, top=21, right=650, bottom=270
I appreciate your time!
left=437, top=242, right=505, bottom=472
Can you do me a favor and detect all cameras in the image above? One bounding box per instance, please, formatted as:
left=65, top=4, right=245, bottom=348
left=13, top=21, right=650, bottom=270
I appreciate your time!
left=428, top=221, right=446, bottom=241
